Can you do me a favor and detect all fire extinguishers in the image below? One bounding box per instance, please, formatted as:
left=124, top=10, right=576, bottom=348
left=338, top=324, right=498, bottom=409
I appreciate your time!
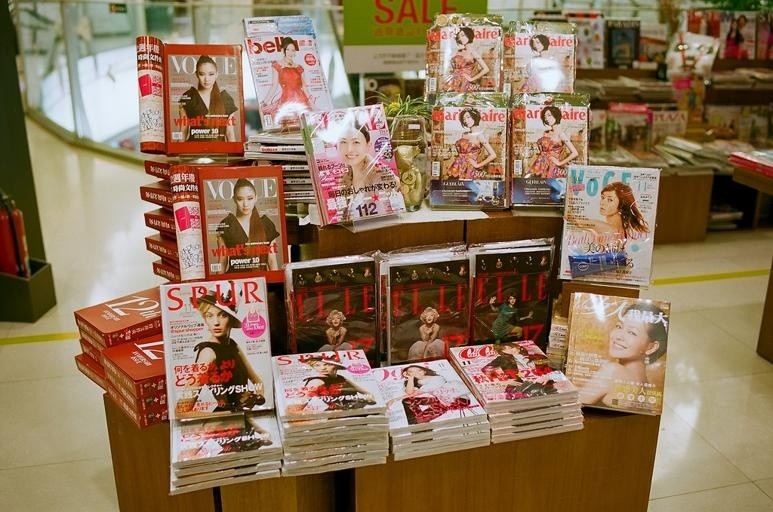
left=0, top=189, right=30, bottom=279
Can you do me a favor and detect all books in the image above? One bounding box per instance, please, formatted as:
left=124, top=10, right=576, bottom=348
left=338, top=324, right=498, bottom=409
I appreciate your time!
left=485, top=0, right=773, bottom=174
left=73, top=14, right=669, bottom=496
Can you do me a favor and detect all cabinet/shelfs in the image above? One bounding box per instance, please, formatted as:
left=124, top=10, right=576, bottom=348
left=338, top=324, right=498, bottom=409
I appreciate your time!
left=103, top=391, right=218, bottom=512
left=355, top=412, right=660, bottom=512
left=217, top=478, right=337, bottom=512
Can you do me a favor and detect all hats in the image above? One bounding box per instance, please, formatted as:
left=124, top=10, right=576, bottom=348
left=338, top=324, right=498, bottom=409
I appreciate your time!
left=298, top=352, right=346, bottom=370
left=190, top=281, right=243, bottom=328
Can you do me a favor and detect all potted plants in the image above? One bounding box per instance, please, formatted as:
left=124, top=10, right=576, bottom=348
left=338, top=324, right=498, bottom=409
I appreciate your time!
left=364, top=91, right=433, bottom=140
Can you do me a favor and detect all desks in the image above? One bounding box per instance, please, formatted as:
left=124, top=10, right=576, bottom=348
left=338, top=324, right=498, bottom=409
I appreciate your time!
left=733, top=166, right=773, bottom=364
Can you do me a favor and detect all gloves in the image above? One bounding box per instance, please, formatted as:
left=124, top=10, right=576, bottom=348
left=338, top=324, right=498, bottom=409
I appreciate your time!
left=232, top=394, right=265, bottom=411
left=333, top=391, right=367, bottom=410
left=231, top=431, right=261, bottom=451
